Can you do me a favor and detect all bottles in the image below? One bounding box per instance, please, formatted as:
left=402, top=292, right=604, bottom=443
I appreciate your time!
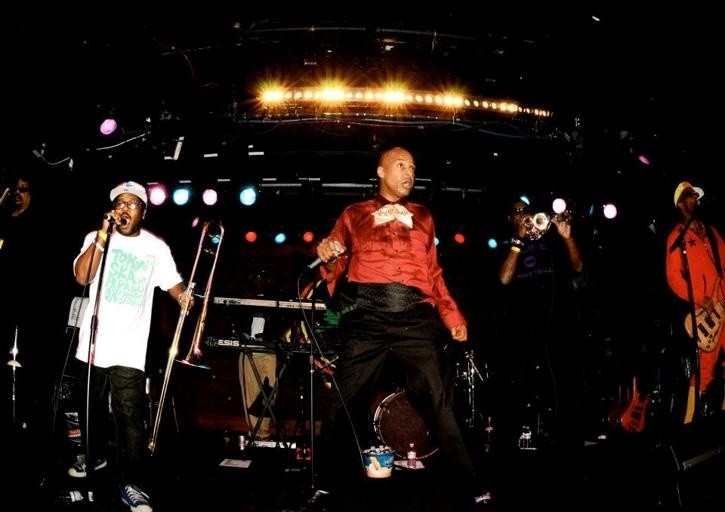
left=239, top=435, right=247, bottom=452
left=224, top=429, right=231, bottom=447
left=407, top=443, right=417, bottom=470
left=367, top=445, right=391, bottom=454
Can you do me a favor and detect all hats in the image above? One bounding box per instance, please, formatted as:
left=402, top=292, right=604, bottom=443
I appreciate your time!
left=110, top=181, right=147, bottom=204
left=674, top=182, right=704, bottom=205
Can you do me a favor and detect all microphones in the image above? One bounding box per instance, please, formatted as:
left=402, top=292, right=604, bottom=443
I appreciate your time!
left=105, top=214, right=129, bottom=226
left=307, top=240, right=342, bottom=270
left=552, top=214, right=573, bottom=223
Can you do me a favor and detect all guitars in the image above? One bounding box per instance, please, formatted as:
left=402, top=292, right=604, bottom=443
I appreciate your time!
left=618, top=375, right=661, bottom=434
left=684, top=276, right=723, bottom=353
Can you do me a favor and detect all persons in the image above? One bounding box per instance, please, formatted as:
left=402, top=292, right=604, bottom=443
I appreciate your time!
left=0, top=146, right=725, bottom=511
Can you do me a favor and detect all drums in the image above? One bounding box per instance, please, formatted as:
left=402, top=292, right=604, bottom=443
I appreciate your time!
left=371, top=388, right=439, bottom=460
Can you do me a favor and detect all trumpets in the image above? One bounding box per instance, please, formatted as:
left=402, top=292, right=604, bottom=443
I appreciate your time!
left=522, top=210, right=572, bottom=240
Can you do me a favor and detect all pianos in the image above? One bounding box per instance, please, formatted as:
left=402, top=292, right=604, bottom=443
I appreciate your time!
left=204, top=296, right=334, bottom=355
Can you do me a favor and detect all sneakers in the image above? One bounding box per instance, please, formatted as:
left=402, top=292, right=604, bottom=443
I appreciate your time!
left=120, top=484, right=152, bottom=512
left=69, top=457, right=106, bottom=477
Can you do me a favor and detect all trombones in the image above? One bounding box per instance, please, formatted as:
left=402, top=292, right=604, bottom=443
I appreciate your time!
left=147, top=220, right=226, bottom=458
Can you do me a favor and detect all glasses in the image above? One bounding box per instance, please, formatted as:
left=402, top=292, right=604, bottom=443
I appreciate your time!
left=514, top=206, right=529, bottom=215
left=115, top=200, right=139, bottom=210
